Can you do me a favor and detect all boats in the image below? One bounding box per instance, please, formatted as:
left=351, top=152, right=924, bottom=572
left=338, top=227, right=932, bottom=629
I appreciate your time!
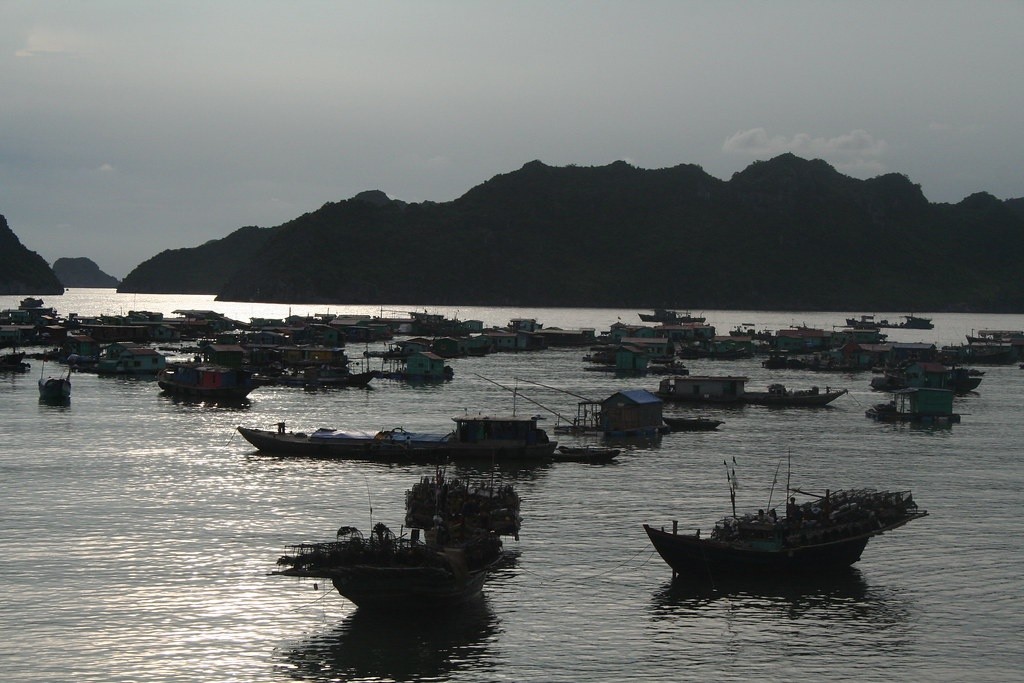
left=236, top=418, right=558, bottom=460
left=643, top=447, right=930, bottom=573
left=553, top=444, right=621, bottom=464
left=0, top=294, right=997, bottom=436
left=263, top=465, right=524, bottom=614
